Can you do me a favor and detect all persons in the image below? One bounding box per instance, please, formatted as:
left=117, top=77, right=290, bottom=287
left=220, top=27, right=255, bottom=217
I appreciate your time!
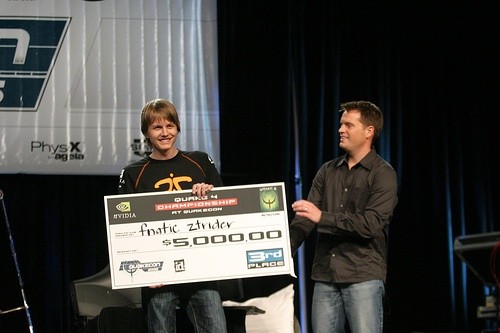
left=288, top=101, right=397, bottom=333
left=116, top=98, right=233, bottom=333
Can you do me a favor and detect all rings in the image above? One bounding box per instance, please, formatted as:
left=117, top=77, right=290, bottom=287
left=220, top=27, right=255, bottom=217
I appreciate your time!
left=304, top=207, right=308, bottom=212
